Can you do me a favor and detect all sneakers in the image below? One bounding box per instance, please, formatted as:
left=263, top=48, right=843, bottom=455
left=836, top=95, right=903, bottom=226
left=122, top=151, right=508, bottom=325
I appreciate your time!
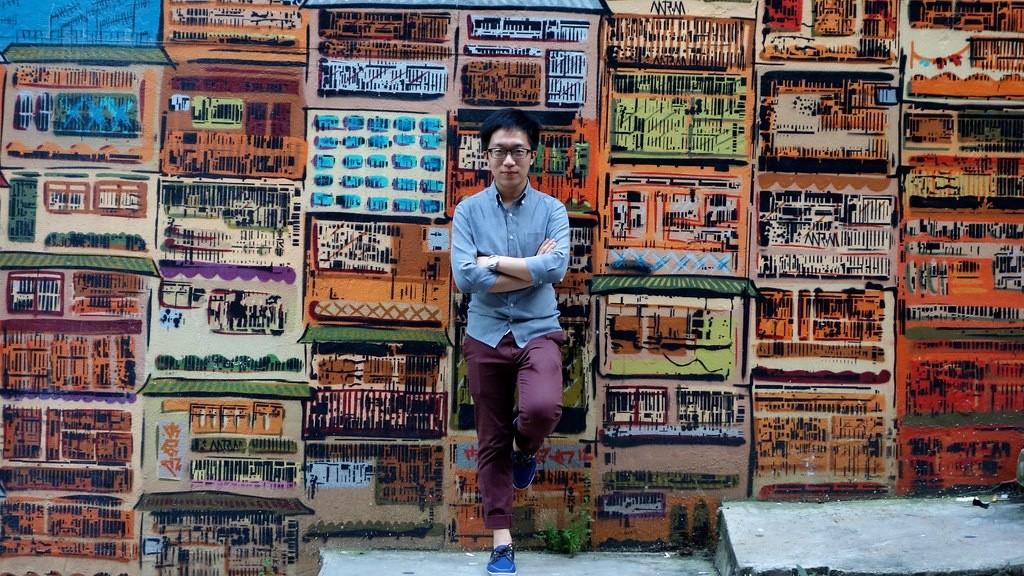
left=512, top=448, right=536, bottom=490
left=486, top=543, right=517, bottom=576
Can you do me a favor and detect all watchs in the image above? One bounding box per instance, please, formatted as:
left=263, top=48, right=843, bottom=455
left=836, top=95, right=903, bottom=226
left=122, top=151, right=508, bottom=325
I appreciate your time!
left=487, top=254, right=499, bottom=271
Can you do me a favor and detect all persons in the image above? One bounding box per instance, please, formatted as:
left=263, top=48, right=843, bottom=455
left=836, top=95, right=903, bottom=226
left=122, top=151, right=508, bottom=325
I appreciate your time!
left=448, top=105, right=573, bottom=576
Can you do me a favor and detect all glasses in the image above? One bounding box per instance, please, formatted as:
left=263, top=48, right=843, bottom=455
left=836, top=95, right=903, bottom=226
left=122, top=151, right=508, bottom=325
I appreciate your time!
left=488, top=148, right=532, bottom=159
